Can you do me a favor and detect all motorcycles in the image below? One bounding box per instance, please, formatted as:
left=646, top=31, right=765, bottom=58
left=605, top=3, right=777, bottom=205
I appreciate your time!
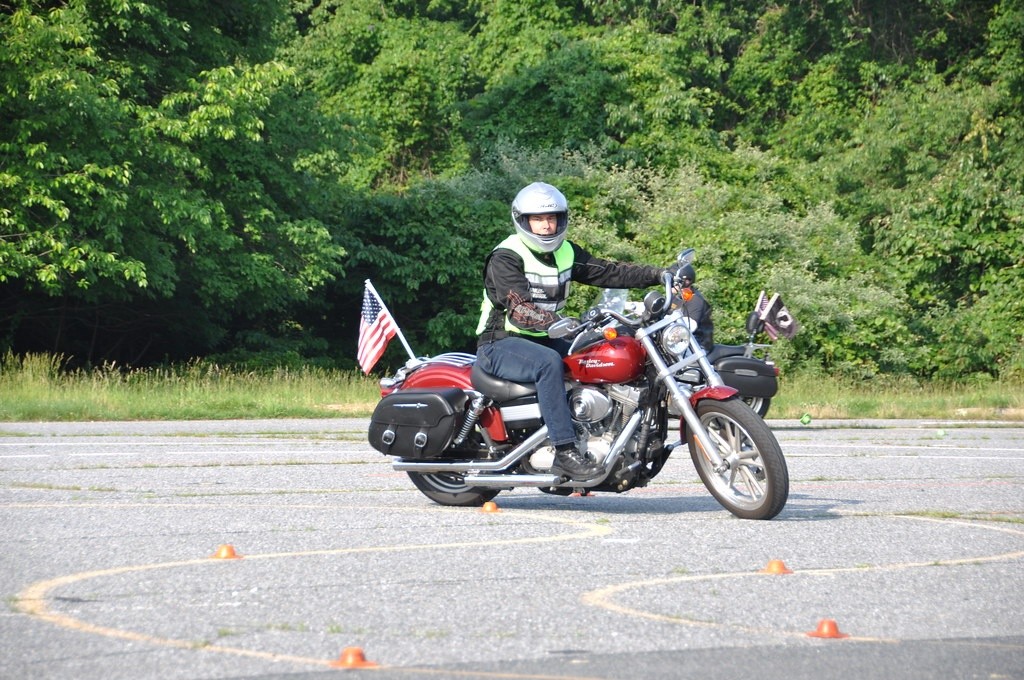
left=365, top=247, right=791, bottom=520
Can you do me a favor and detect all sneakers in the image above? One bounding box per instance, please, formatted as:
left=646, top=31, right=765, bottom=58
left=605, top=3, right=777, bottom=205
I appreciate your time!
left=550, top=448, right=605, bottom=481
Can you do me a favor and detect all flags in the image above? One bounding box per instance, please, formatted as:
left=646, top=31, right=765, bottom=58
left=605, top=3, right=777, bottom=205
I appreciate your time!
left=352, top=278, right=401, bottom=376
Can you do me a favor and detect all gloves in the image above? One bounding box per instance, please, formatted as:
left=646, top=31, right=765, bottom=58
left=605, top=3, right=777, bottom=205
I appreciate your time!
left=561, top=316, right=583, bottom=341
left=661, top=267, right=679, bottom=286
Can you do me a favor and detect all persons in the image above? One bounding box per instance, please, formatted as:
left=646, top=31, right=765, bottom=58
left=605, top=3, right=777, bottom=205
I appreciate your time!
left=474, top=182, right=696, bottom=482
left=666, top=262, right=715, bottom=358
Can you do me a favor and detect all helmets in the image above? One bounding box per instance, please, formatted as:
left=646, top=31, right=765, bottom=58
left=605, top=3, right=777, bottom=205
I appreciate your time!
left=670, top=262, right=695, bottom=288
left=511, top=182, right=568, bottom=254
